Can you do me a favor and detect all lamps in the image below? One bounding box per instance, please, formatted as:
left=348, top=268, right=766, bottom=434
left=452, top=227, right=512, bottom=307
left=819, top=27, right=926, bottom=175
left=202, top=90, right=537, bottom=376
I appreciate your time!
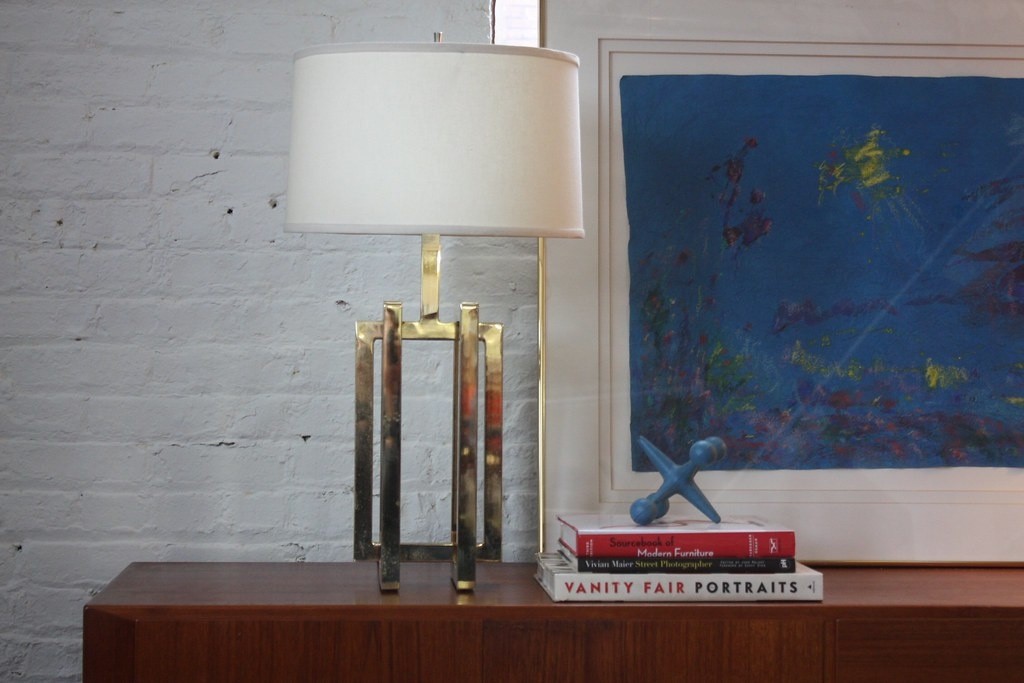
left=278, top=31, right=584, bottom=589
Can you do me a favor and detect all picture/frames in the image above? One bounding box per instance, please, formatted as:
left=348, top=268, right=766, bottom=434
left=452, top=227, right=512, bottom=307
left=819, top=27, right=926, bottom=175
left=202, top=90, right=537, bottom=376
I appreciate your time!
left=537, top=0, right=1024, bottom=567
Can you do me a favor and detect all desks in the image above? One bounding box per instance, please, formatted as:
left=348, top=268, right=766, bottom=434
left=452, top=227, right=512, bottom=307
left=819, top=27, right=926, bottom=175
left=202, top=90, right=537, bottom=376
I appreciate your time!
left=81, top=557, right=1024, bottom=683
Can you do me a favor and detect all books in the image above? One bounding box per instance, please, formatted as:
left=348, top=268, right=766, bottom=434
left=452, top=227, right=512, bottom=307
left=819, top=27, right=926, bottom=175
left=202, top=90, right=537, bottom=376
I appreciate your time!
left=558, top=514, right=796, bottom=558
left=534, top=553, right=824, bottom=603
left=558, top=550, right=799, bottom=573
